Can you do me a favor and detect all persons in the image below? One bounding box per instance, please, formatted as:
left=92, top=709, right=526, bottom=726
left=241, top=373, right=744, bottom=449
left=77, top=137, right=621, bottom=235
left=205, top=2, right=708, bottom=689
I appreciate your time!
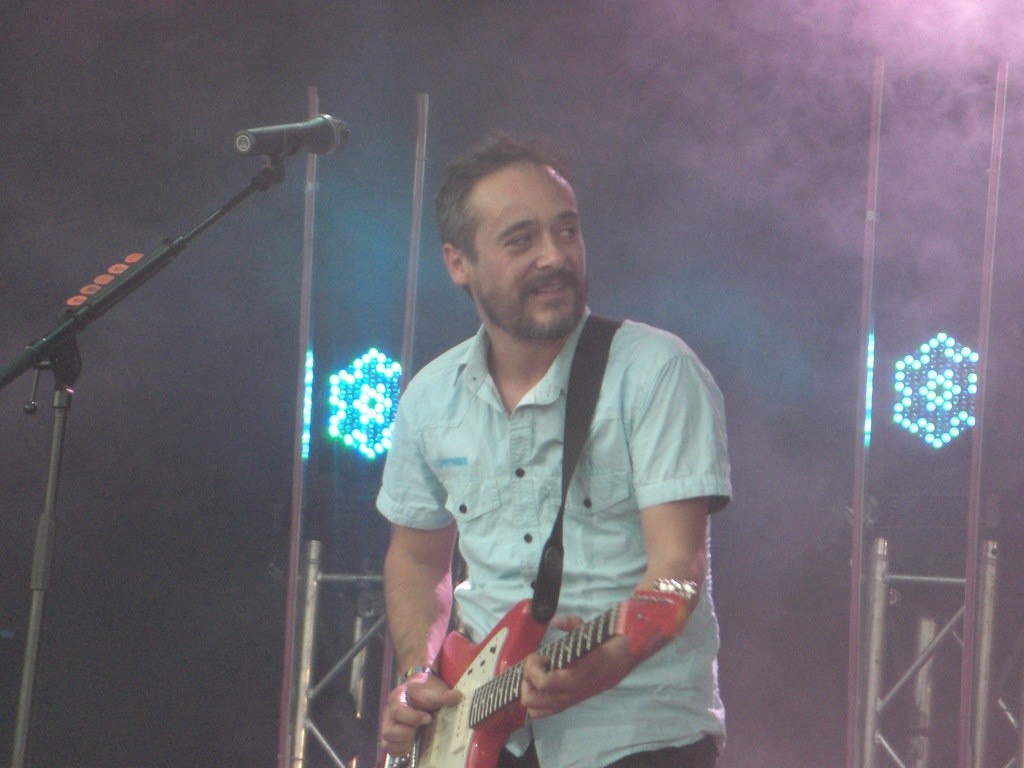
left=379, top=139, right=734, bottom=768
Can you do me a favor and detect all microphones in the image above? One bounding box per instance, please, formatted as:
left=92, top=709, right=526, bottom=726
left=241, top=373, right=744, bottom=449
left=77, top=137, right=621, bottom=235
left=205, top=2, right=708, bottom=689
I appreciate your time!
left=233, top=113, right=349, bottom=156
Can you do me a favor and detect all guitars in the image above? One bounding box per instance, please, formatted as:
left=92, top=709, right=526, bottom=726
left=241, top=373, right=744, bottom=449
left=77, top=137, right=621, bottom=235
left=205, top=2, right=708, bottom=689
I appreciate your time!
left=358, top=577, right=701, bottom=768
